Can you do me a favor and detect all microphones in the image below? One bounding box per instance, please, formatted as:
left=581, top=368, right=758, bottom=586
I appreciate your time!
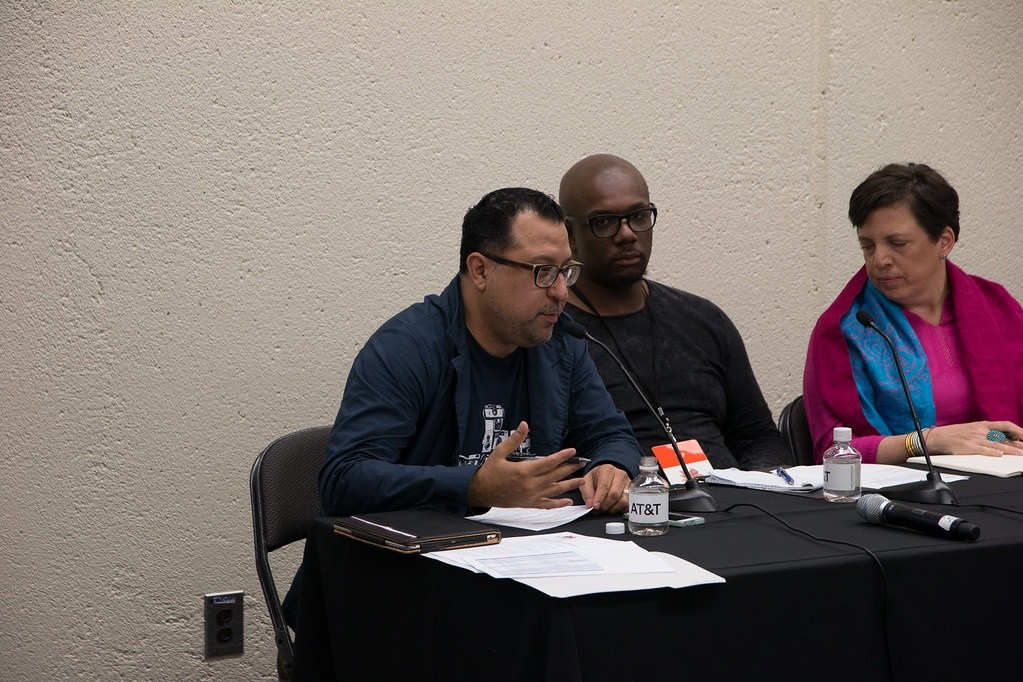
left=856, top=492, right=980, bottom=543
left=562, top=320, right=719, bottom=513
left=855, top=310, right=958, bottom=507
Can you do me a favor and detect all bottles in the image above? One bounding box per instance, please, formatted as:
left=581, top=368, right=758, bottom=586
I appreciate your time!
left=628, top=457, right=670, bottom=537
left=822, top=427, right=861, bottom=504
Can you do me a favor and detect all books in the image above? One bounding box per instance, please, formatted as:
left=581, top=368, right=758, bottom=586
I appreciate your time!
left=331, top=509, right=503, bottom=555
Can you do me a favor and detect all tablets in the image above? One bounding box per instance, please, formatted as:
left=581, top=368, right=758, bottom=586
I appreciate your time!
left=334, top=512, right=501, bottom=555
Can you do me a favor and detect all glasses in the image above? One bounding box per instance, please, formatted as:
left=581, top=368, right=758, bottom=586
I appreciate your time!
left=564, top=203, right=657, bottom=238
left=479, top=251, right=584, bottom=289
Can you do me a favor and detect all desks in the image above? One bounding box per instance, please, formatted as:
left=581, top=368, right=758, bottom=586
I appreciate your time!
left=287, top=457, right=1023, bottom=682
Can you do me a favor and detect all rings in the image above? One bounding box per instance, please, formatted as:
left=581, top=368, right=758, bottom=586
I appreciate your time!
left=623, top=488, right=629, bottom=495
left=987, top=430, right=1007, bottom=443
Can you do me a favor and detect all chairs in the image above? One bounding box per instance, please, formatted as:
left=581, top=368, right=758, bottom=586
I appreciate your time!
left=778, top=395, right=817, bottom=466
left=250, top=425, right=331, bottom=682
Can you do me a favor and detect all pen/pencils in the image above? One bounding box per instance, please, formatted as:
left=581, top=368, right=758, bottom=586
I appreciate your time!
left=506, top=455, right=592, bottom=464
left=777, top=465, right=795, bottom=485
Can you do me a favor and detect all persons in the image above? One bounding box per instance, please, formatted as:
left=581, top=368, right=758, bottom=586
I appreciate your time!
left=802, top=163, right=1023, bottom=463
left=278, top=186, right=646, bottom=682
left=558, top=154, right=794, bottom=473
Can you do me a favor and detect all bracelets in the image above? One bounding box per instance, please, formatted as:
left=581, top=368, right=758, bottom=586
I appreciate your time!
left=905, top=425, right=932, bottom=456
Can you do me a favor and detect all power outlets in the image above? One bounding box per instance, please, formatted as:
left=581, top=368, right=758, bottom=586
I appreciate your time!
left=205, top=590, right=243, bottom=657
